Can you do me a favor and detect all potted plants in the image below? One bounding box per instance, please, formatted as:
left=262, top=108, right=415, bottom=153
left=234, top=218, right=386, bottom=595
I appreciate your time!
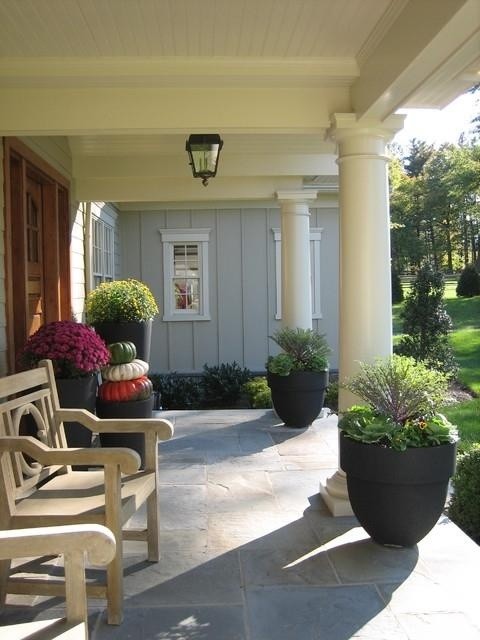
left=338, top=353, right=460, bottom=550
left=85, top=275, right=160, bottom=371
left=265, top=325, right=332, bottom=432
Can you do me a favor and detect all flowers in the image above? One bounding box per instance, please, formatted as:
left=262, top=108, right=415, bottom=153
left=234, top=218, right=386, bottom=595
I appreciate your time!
left=16, top=317, right=112, bottom=379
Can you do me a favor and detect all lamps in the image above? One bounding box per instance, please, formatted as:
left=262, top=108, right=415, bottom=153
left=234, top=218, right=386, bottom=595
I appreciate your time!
left=185, top=131, right=224, bottom=187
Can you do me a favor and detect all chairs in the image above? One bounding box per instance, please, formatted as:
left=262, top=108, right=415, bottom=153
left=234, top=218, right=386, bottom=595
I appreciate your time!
left=0, top=360, right=173, bottom=640
